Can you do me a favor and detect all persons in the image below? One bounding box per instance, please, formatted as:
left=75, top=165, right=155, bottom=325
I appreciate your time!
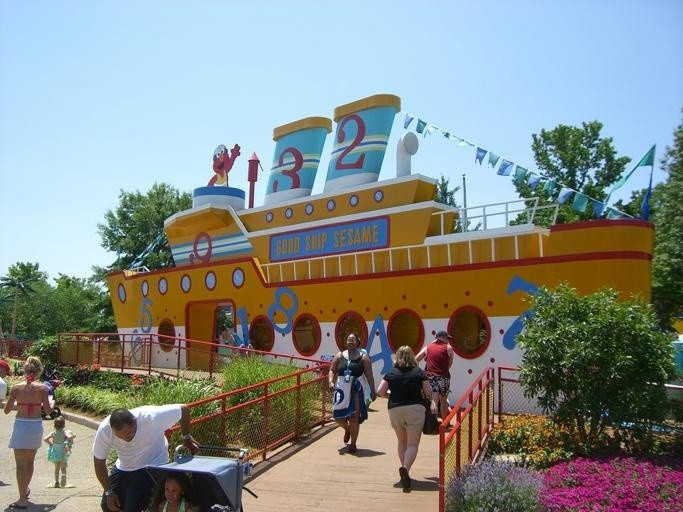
left=0, top=360, right=12, bottom=486
left=415, top=331, right=453, bottom=427
left=93, top=404, right=199, bottom=512
left=376, top=346, right=433, bottom=488
left=44, top=416, right=73, bottom=488
left=156, top=472, right=202, bottom=512
left=329, top=333, right=376, bottom=454
left=220, top=325, right=242, bottom=347
left=4, top=357, right=55, bottom=509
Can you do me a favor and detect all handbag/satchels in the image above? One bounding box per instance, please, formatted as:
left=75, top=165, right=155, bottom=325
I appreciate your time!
left=422, top=393, right=438, bottom=436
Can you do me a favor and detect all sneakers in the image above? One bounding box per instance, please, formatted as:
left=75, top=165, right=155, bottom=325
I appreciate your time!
left=399, top=467, right=410, bottom=489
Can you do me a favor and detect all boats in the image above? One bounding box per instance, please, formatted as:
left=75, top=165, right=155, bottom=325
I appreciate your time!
left=105, top=95, right=658, bottom=419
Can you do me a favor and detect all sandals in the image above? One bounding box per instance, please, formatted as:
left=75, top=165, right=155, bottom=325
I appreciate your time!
left=349, top=444, right=356, bottom=452
left=344, top=431, right=349, bottom=443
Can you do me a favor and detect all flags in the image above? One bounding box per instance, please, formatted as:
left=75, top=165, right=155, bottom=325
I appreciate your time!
left=404, top=113, right=634, bottom=220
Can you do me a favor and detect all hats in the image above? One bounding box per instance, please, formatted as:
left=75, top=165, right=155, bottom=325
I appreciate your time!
left=435, top=331, right=452, bottom=340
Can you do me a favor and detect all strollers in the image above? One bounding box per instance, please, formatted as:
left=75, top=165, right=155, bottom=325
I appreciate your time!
left=125, top=439, right=252, bottom=511
left=35, top=360, right=62, bottom=418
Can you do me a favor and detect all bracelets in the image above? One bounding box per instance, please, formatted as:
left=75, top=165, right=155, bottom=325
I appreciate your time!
left=181, top=432, right=192, bottom=440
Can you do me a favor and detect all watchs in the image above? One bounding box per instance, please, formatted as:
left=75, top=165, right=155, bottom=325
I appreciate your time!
left=104, top=488, right=115, bottom=497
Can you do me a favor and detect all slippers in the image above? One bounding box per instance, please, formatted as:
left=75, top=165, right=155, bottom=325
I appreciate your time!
left=26, top=488, right=29, bottom=497
left=9, top=502, right=26, bottom=509
